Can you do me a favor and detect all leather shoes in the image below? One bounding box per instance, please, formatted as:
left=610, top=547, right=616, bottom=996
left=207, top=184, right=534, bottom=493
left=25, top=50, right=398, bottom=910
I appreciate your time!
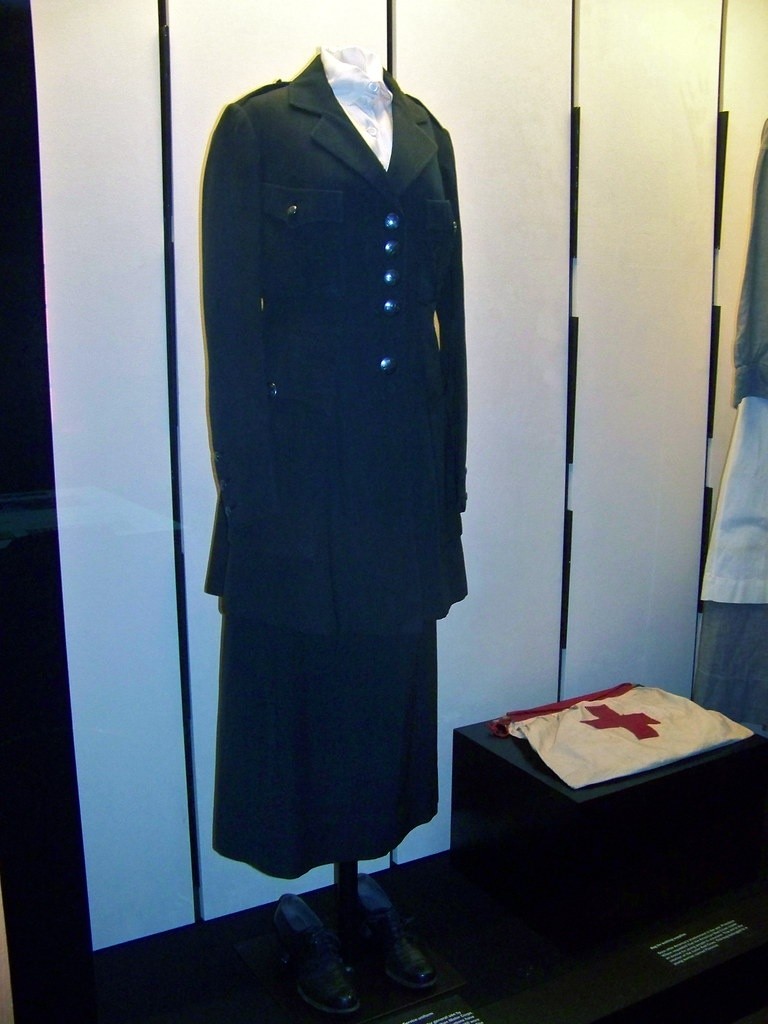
left=266, top=893, right=360, bottom=1012
left=356, top=873, right=438, bottom=988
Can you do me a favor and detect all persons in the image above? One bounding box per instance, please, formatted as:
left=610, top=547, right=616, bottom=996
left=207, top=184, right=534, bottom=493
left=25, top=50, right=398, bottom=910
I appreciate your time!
left=204, top=48, right=469, bottom=884
